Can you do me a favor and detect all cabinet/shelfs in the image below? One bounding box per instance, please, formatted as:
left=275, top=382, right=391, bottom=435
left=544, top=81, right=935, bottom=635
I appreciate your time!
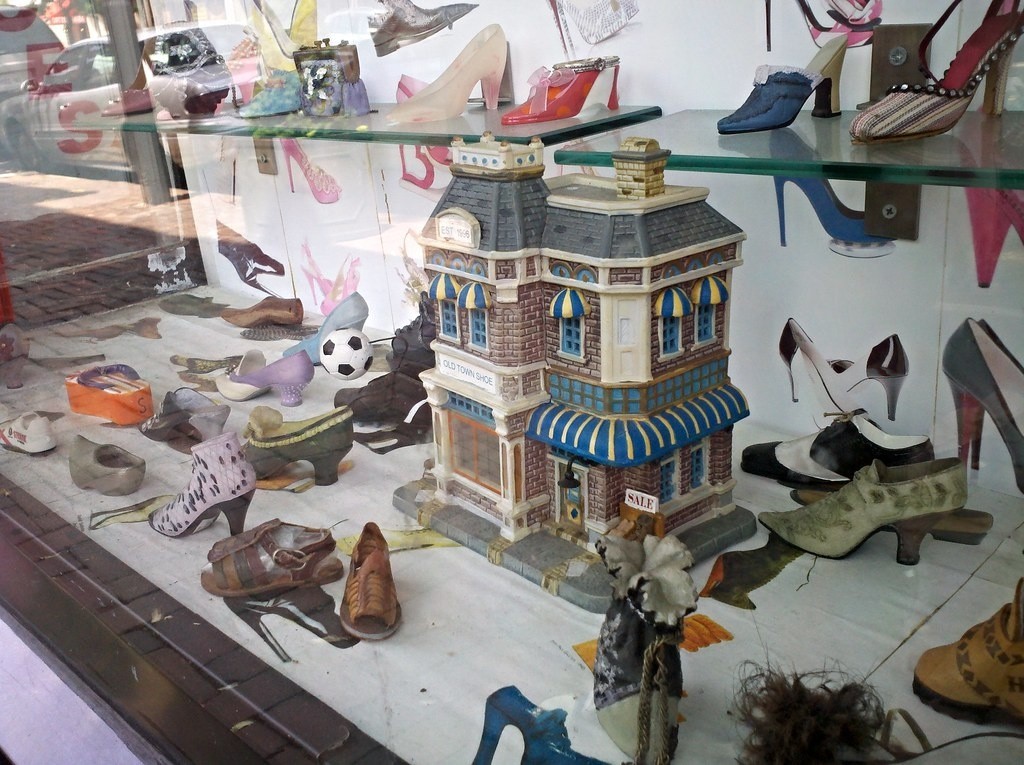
left=0, top=0, right=1024, bottom=765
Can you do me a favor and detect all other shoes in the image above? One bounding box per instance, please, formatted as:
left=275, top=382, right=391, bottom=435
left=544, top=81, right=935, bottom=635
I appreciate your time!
left=0, top=291, right=437, bottom=641
left=740, top=409, right=1024, bottom=726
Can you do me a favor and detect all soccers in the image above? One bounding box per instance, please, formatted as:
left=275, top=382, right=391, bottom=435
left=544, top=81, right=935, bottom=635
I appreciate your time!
left=320, top=328, right=374, bottom=381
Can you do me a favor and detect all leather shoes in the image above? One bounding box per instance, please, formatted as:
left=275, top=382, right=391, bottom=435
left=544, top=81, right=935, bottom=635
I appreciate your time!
left=367, top=0, right=479, bottom=56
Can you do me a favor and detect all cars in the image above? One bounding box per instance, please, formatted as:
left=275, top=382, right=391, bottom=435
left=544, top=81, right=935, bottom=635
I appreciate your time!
left=0, top=20, right=268, bottom=192
left=0, top=4, right=67, bottom=162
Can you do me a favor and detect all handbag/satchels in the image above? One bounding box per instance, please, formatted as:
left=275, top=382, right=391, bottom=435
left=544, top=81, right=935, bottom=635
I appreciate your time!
left=294, top=37, right=370, bottom=118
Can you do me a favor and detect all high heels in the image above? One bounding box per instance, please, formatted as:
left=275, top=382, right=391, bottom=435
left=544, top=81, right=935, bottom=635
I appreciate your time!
left=279, top=135, right=343, bottom=203
left=769, top=128, right=897, bottom=246
left=766, top=0, right=883, bottom=51
left=544, top=0, right=640, bottom=59
left=963, top=187, right=1024, bottom=288
left=472, top=684, right=610, bottom=764
left=500, top=54, right=621, bottom=125
left=716, top=33, right=848, bottom=132
left=385, top=23, right=506, bottom=123
left=250, top=0, right=301, bottom=72
left=103, top=27, right=303, bottom=120
left=942, top=316, right=1024, bottom=493
left=779, top=316, right=909, bottom=424
left=299, top=240, right=361, bottom=317
left=848, top=0, right=1024, bottom=142
